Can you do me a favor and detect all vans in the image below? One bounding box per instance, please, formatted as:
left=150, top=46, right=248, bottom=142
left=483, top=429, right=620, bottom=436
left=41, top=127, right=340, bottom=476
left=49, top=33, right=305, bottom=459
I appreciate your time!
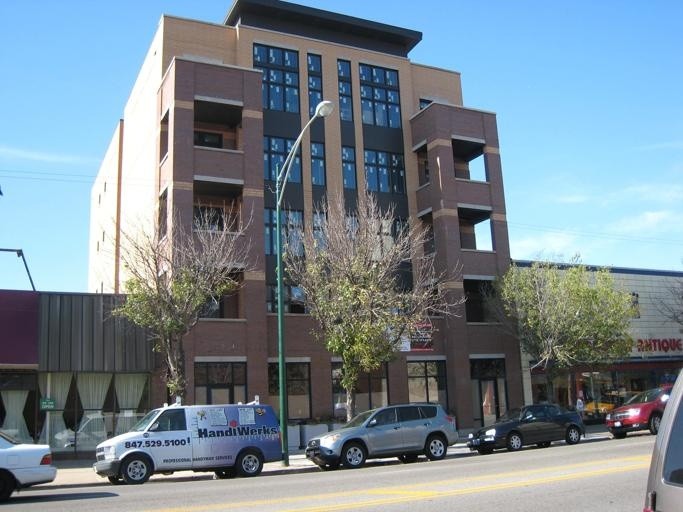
left=55, top=407, right=146, bottom=454
left=91, top=401, right=282, bottom=484
left=642, top=369, right=682, bottom=512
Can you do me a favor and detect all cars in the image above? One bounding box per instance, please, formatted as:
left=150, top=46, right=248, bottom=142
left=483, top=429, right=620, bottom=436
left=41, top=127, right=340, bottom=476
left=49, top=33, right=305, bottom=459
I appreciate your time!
left=469, top=402, right=586, bottom=453
left=0, top=429, right=55, bottom=504
left=583, top=388, right=639, bottom=419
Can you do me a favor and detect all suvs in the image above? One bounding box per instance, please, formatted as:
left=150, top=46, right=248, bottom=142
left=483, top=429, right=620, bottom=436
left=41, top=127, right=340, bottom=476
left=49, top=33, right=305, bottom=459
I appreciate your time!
left=606, top=384, right=674, bottom=438
left=303, top=400, right=456, bottom=472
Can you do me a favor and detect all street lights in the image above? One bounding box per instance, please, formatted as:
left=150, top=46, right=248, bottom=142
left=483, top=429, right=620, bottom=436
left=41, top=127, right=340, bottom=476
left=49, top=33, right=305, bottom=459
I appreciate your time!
left=272, top=99, right=335, bottom=467
